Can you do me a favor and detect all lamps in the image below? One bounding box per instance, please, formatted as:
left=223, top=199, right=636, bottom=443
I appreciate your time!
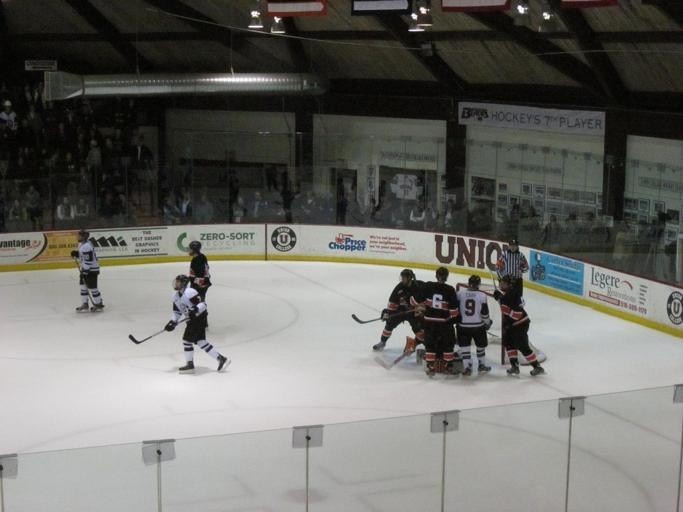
left=416, top=7, right=433, bottom=27
left=513, top=4, right=532, bottom=26
left=538, top=10, right=558, bottom=34
left=248, top=10, right=264, bottom=29
left=408, top=13, right=425, bottom=32
left=270, top=16, right=287, bottom=33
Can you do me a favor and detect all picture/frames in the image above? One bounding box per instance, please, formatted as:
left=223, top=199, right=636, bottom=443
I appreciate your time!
left=495, top=182, right=680, bottom=242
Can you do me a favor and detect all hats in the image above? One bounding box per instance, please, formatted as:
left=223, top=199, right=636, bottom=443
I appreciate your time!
left=508, top=240, right=519, bottom=245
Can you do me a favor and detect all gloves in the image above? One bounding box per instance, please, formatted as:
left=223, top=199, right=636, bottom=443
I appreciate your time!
left=188, top=305, right=199, bottom=321
left=71, top=250, right=79, bottom=259
left=164, top=320, right=177, bottom=332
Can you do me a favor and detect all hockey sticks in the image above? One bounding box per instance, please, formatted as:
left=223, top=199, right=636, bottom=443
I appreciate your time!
left=487, top=243, right=497, bottom=291
left=129, top=317, right=189, bottom=344
left=373, top=346, right=417, bottom=369
left=73, top=256, right=105, bottom=310
left=351, top=309, right=416, bottom=323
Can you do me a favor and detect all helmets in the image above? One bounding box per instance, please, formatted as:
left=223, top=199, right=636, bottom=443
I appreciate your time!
left=399, top=267, right=517, bottom=292
left=78, top=231, right=89, bottom=242
left=189, top=241, right=201, bottom=256
left=173, top=275, right=190, bottom=291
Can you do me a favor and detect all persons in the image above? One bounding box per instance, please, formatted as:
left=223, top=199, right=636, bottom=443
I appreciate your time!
left=166, top=274, right=227, bottom=371
left=189, top=240, right=211, bottom=327
left=410, top=267, right=462, bottom=377
left=495, top=275, right=544, bottom=376
left=71, top=229, right=104, bottom=312
left=0, top=79, right=682, bottom=278
left=495, top=238, right=528, bottom=298
left=373, top=269, right=425, bottom=350
left=456, top=274, right=491, bottom=375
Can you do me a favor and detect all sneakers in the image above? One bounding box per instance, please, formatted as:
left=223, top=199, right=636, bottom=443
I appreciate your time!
left=76, top=303, right=89, bottom=310
left=90, top=301, right=105, bottom=311
left=217, top=357, right=226, bottom=370
left=372, top=341, right=544, bottom=377
left=178, top=365, right=195, bottom=371
